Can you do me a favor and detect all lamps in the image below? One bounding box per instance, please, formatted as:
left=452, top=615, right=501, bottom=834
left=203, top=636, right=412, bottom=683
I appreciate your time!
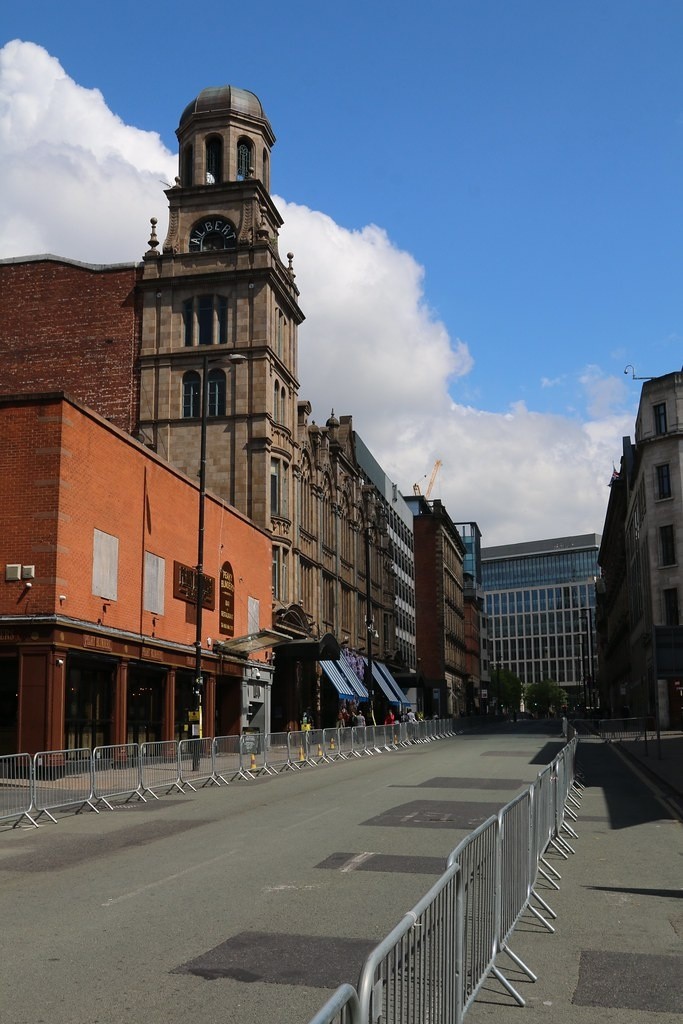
left=251, top=667, right=261, bottom=680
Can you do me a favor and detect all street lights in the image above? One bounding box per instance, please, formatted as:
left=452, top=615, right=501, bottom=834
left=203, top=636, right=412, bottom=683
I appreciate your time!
left=364, top=525, right=387, bottom=727
left=580, top=608, right=596, bottom=711
left=577, top=633, right=587, bottom=709
left=580, top=616, right=591, bottom=709
left=185, top=352, right=248, bottom=767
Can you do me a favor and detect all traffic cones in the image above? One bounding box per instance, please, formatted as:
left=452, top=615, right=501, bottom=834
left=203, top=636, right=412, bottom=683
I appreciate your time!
left=298, top=746, right=306, bottom=763
left=328, top=738, right=336, bottom=750
left=248, top=754, right=259, bottom=774
left=394, top=734, right=399, bottom=744
left=316, top=744, right=324, bottom=757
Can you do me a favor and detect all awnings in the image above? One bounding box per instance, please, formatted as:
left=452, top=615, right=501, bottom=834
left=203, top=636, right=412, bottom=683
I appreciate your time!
left=375, top=661, right=411, bottom=707
left=318, top=660, right=354, bottom=700
left=336, top=650, right=369, bottom=702
left=362, top=657, right=400, bottom=706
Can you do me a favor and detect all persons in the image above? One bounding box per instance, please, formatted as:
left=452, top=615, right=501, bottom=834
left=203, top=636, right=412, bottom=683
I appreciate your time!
left=336, top=708, right=366, bottom=745
left=384, top=708, right=438, bottom=744
left=620, top=704, right=630, bottom=732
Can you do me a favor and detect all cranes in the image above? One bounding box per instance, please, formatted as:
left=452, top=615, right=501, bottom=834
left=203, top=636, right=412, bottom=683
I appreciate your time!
left=413, top=458, right=443, bottom=499
